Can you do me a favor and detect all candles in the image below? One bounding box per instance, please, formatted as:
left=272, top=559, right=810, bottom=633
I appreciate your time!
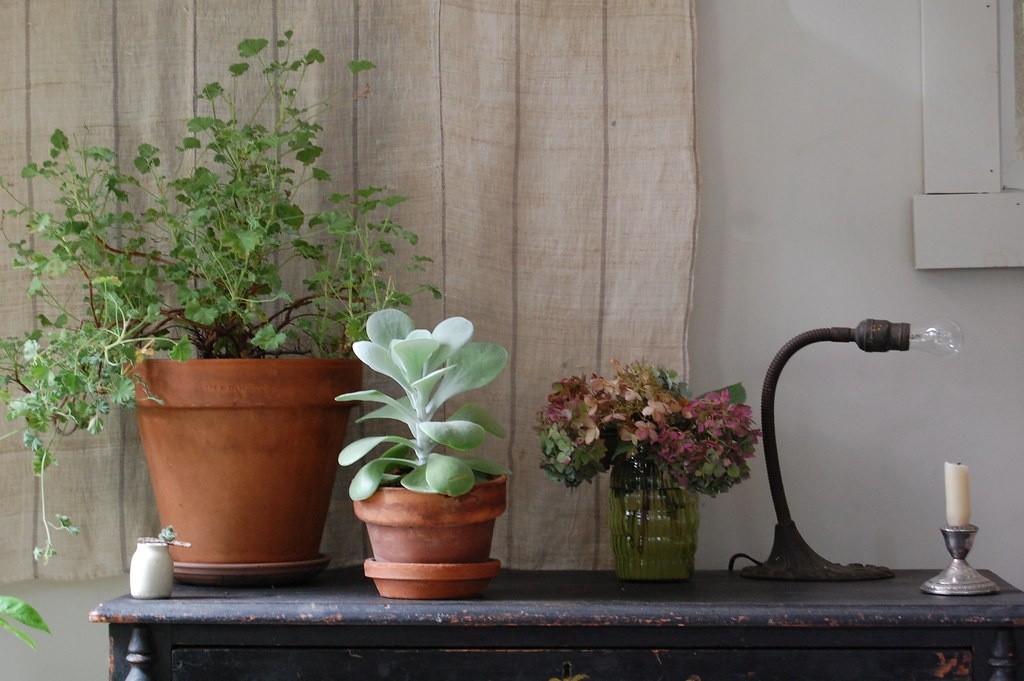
left=945, top=459, right=971, bottom=525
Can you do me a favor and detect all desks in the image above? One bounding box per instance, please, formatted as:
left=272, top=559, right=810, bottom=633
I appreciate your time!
left=89, top=569, right=1024, bottom=681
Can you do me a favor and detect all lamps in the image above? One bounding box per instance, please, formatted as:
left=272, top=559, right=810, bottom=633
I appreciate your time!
left=726, top=318, right=964, bottom=584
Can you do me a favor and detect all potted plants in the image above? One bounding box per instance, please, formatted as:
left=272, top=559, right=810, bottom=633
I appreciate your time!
left=0, top=29, right=444, bottom=587
left=334, top=307, right=513, bottom=600
left=129, top=525, right=193, bottom=599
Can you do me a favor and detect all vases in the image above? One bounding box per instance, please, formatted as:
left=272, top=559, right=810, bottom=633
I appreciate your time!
left=607, top=463, right=698, bottom=581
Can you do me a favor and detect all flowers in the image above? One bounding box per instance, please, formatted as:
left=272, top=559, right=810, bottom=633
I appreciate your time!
left=533, top=357, right=763, bottom=500
